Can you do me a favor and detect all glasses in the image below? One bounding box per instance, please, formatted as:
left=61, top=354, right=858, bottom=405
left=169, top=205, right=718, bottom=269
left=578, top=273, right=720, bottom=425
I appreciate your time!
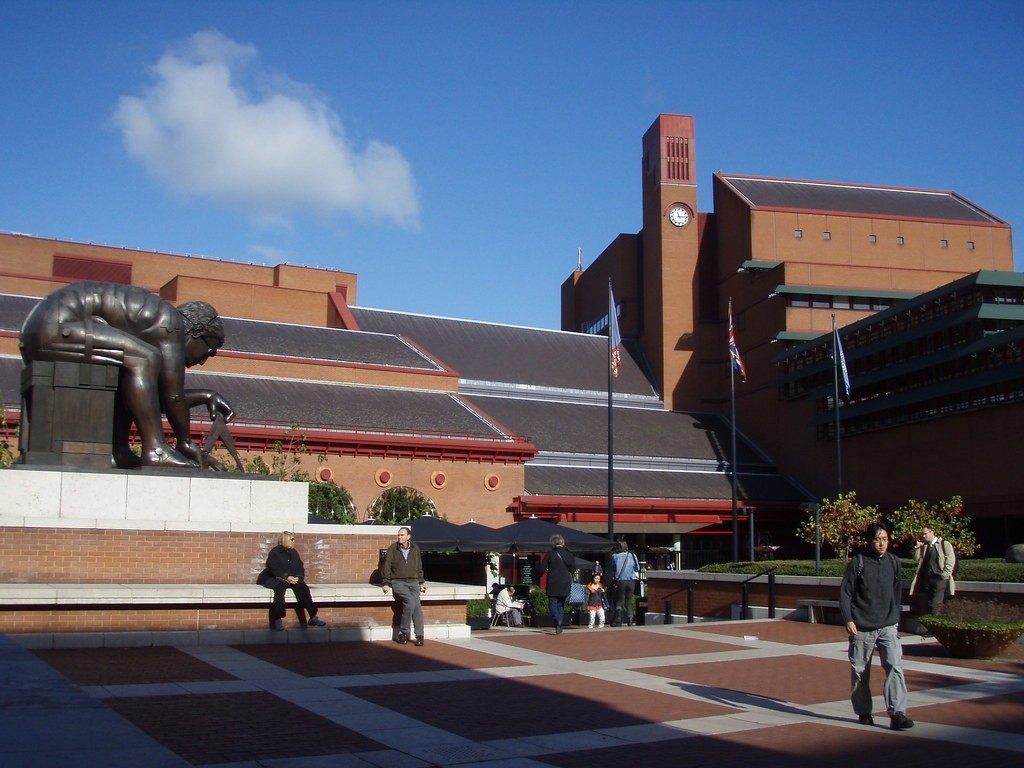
left=290, top=538, right=294, bottom=541
left=921, top=531, right=931, bottom=535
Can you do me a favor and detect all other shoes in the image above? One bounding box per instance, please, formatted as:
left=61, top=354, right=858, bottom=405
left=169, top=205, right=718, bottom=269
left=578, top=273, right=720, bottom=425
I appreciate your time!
left=515, top=624, right=525, bottom=628
left=556, top=620, right=563, bottom=634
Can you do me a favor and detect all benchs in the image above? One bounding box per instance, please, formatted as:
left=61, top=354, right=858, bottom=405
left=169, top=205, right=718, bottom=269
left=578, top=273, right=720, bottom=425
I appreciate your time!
left=796, top=599, right=910, bottom=631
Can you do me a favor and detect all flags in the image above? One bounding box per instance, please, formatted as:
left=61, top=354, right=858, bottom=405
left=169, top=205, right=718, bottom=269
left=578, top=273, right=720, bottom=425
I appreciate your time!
left=835, top=320, right=851, bottom=395
left=611, top=278, right=622, bottom=379
left=728, top=298, right=746, bottom=383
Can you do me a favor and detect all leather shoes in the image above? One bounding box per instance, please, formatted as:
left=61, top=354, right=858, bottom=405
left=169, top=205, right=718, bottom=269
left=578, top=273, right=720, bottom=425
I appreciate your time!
left=610, top=609, right=621, bottom=627
left=628, top=617, right=632, bottom=626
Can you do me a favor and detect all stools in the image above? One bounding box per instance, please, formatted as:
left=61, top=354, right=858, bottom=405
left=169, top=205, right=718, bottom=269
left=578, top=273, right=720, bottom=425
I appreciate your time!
left=490, top=598, right=515, bottom=627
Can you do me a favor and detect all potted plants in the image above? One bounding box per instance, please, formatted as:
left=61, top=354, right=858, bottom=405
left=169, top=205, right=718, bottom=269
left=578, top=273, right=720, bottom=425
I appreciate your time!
left=916, top=598, right=1024, bottom=659
left=466, top=586, right=636, bottom=630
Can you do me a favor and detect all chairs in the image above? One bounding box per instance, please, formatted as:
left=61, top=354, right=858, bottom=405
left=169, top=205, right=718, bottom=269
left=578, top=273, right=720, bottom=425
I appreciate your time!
left=516, top=599, right=532, bottom=626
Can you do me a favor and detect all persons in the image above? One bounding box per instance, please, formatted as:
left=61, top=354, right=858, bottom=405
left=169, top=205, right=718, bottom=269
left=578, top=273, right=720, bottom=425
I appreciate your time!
left=608, top=540, right=640, bottom=627
left=21, top=281, right=235, bottom=468
left=495, top=587, right=525, bottom=628
left=381, top=527, right=427, bottom=646
left=909, top=525, right=956, bottom=637
left=572, top=560, right=603, bottom=584
left=537, top=534, right=575, bottom=634
left=586, top=573, right=605, bottom=628
left=265, top=531, right=326, bottom=631
left=838, top=521, right=914, bottom=730
left=646, top=553, right=676, bottom=570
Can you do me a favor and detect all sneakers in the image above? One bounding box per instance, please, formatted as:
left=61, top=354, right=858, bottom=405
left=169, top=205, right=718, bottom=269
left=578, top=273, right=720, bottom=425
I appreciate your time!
left=859, top=713, right=873, bottom=725
left=890, top=711, right=914, bottom=730
left=416, top=635, right=424, bottom=646
left=269, top=618, right=284, bottom=631
left=398, top=631, right=407, bottom=644
left=308, top=616, right=326, bottom=626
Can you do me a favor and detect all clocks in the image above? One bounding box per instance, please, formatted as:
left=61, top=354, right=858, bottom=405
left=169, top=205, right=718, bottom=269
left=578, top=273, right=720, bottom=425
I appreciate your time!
left=669, top=207, right=689, bottom=227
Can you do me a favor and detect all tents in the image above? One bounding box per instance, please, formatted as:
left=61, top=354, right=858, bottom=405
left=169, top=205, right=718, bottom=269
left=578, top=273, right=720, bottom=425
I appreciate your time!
left=309, top=510, right=618, bottom=584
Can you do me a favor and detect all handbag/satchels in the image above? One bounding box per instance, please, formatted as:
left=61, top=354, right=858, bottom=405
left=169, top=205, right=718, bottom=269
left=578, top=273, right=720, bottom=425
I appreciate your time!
left=566, top=584, right=584, bottom=606
left=610, top=578, right=619, bottom=600
left=941, top=540, right=959, bottom=574
